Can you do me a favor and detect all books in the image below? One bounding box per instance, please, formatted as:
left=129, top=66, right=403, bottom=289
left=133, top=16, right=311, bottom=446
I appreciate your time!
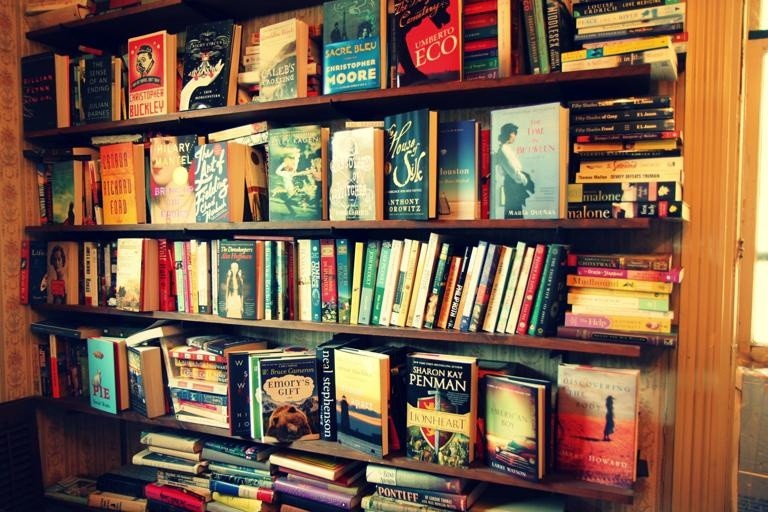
left=18, top=0, right=691, bottom=511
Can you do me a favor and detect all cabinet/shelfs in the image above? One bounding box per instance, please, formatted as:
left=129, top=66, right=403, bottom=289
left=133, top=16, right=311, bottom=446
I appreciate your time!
left=25, top=0, right=686, bottom=512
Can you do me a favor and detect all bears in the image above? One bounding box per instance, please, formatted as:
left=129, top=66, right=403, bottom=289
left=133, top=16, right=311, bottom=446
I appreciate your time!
left=266, top=405, right=311, bottom=445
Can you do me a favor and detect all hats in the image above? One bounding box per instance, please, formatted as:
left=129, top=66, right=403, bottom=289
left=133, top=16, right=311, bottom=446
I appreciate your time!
left=137, top=46, right=152, bottom=55
